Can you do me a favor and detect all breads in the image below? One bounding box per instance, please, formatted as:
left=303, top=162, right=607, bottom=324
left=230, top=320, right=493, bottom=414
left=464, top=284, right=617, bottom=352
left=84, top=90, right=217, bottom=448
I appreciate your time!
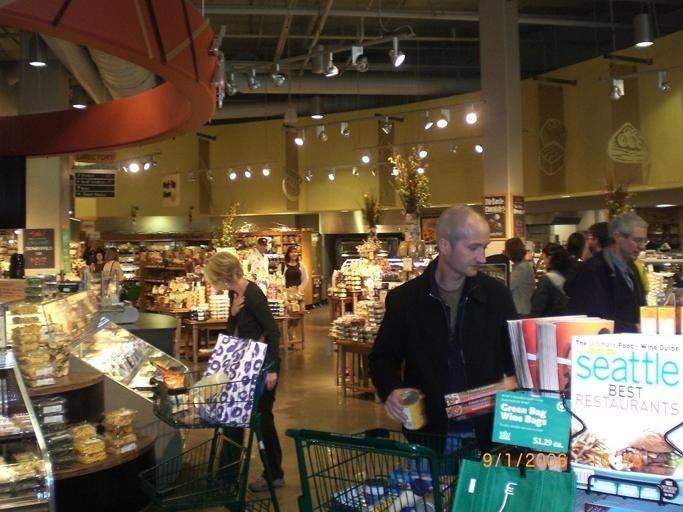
left=1, top=299, right=71, bottom=390
left=0, top=396, right=139, bottom=484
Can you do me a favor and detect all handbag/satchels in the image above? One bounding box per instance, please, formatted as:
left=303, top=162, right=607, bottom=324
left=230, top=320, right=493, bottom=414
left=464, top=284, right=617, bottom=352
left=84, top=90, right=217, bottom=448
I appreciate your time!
left=197, top=333, right=268, bottom=427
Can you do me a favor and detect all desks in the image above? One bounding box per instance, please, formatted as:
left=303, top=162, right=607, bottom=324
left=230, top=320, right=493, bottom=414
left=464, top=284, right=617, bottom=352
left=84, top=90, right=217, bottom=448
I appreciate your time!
left=185, top=314, right=292, bottom=364
left=152, top=306, right=188, bottom=361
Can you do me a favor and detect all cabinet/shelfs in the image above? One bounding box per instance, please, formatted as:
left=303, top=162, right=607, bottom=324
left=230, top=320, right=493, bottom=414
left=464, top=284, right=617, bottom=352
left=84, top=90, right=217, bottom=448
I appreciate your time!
left=24, top=360, right=182, bottom=511
left=0, top=344, right=58, bottom=512
left=41, top=290, right=194, bottom=483
left=250, top=232, right=322, bottom=305
left=134, top=262, right=188, bottom=311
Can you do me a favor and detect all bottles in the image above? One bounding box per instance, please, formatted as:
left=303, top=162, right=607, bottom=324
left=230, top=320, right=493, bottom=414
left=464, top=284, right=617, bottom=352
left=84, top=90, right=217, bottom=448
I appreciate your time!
left=388, top=479, right=429, bottom=512
left=364, top=478, right=388, bottom=506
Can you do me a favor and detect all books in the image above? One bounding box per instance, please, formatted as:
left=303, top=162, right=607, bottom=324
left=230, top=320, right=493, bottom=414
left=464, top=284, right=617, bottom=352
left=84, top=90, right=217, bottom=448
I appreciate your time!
left=505, top=306, right=683, bottom=507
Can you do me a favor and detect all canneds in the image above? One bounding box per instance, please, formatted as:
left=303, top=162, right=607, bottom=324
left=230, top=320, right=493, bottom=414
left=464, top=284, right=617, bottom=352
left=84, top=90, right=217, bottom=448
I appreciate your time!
left=398, top=389, right=425, bottom=432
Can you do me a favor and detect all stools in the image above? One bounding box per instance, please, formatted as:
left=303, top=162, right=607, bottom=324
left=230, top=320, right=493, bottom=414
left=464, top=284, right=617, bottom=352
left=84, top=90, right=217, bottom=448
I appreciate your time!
left=328, top=295, right=352, bottom=319
left=286, top=310, right=306, bottom=349
left=335, top=338, right=378, bottom=402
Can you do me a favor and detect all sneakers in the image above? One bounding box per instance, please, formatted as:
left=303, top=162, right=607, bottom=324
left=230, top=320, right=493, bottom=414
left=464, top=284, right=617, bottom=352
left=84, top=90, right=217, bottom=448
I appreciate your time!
left=249, top=476, right=284, bottom=492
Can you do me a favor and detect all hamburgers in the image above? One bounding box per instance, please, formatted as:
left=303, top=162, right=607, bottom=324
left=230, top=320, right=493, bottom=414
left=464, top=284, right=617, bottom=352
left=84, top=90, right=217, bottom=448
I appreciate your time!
left=620, top=433, right=677, bottom=478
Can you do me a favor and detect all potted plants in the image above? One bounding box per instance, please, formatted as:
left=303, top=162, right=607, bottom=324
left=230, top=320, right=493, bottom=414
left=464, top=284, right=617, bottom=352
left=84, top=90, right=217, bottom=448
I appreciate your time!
left=354, top=191, right=385, bottom=241
left=385, top=142, right=432, bottom=241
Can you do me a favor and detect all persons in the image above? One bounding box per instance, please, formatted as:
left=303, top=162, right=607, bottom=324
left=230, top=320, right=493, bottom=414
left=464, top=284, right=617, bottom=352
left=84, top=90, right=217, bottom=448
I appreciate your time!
left=575, top=211, right=650, bottom=333
left=368, top=205, right=523, bottom=475
left=204, top=252, right=284, bottom=493
left=83, top=244, right=127, bottom=295
left=504, top=222, right=610, bottom=316
left=248, top=238, right=269, bottom=274
left=278, top=246, right=309, bottom=348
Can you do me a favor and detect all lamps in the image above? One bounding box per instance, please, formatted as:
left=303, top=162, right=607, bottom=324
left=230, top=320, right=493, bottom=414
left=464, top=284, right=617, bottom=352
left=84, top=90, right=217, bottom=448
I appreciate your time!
left=630, top=13, right=655, bottom=47
left=222, top=30, right=419, bottom=100
left=71, top=86, right=87, bottom=109
left=607, top=0, right=623, bottom=100
left=28, top=37, right=47, bottom=67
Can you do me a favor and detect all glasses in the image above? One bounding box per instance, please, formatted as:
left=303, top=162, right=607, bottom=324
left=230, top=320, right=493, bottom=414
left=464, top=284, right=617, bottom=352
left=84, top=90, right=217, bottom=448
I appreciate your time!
left=624, top=232, right=650, bottom=246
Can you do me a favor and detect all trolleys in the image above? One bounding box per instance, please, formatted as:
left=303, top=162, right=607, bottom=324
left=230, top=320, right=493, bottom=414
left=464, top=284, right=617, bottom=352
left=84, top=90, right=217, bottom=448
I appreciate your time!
left=135, top=368, right=281, bottom=511
left=283, top=425, right=523, bottom=511
